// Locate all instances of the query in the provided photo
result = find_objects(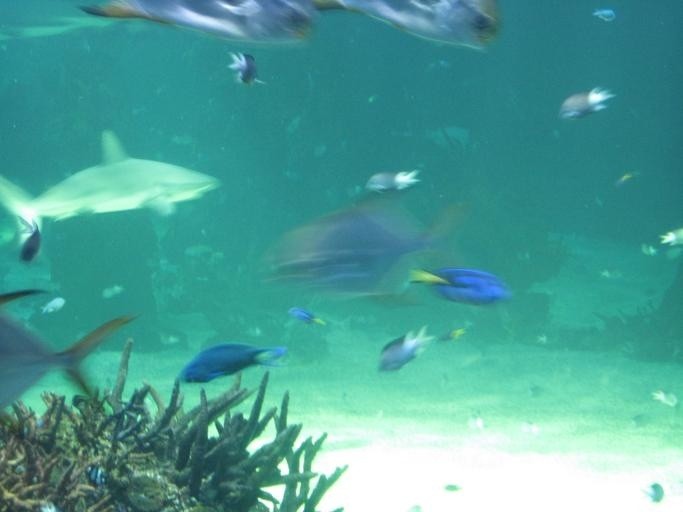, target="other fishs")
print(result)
[81,0,501,49]
[265,210,516,378]
